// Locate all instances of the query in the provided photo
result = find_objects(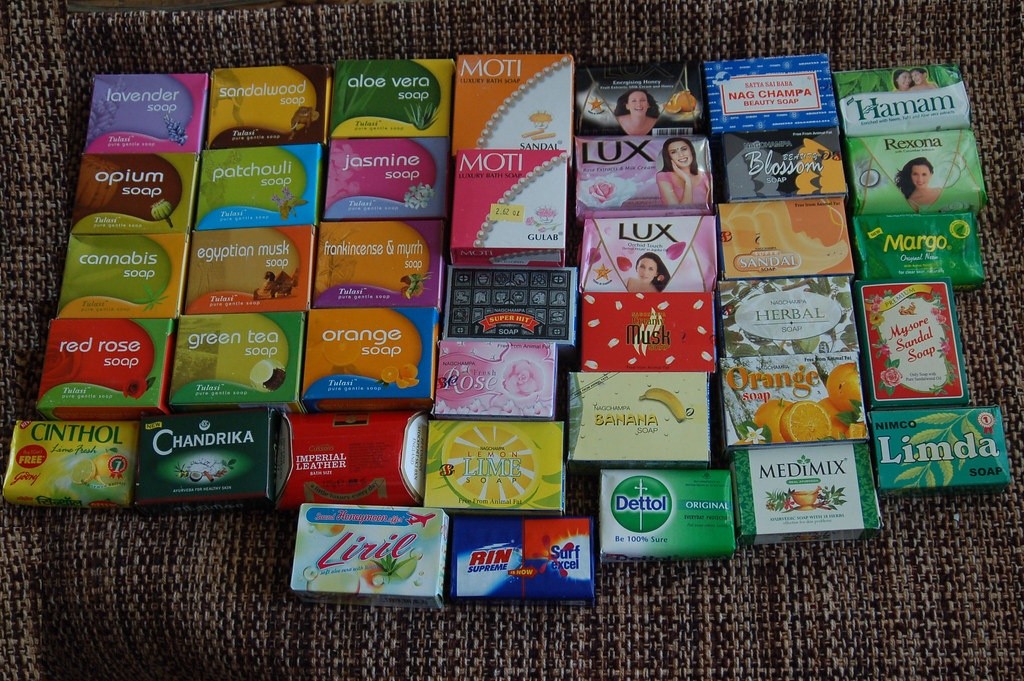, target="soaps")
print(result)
[2,52,1014,609]
